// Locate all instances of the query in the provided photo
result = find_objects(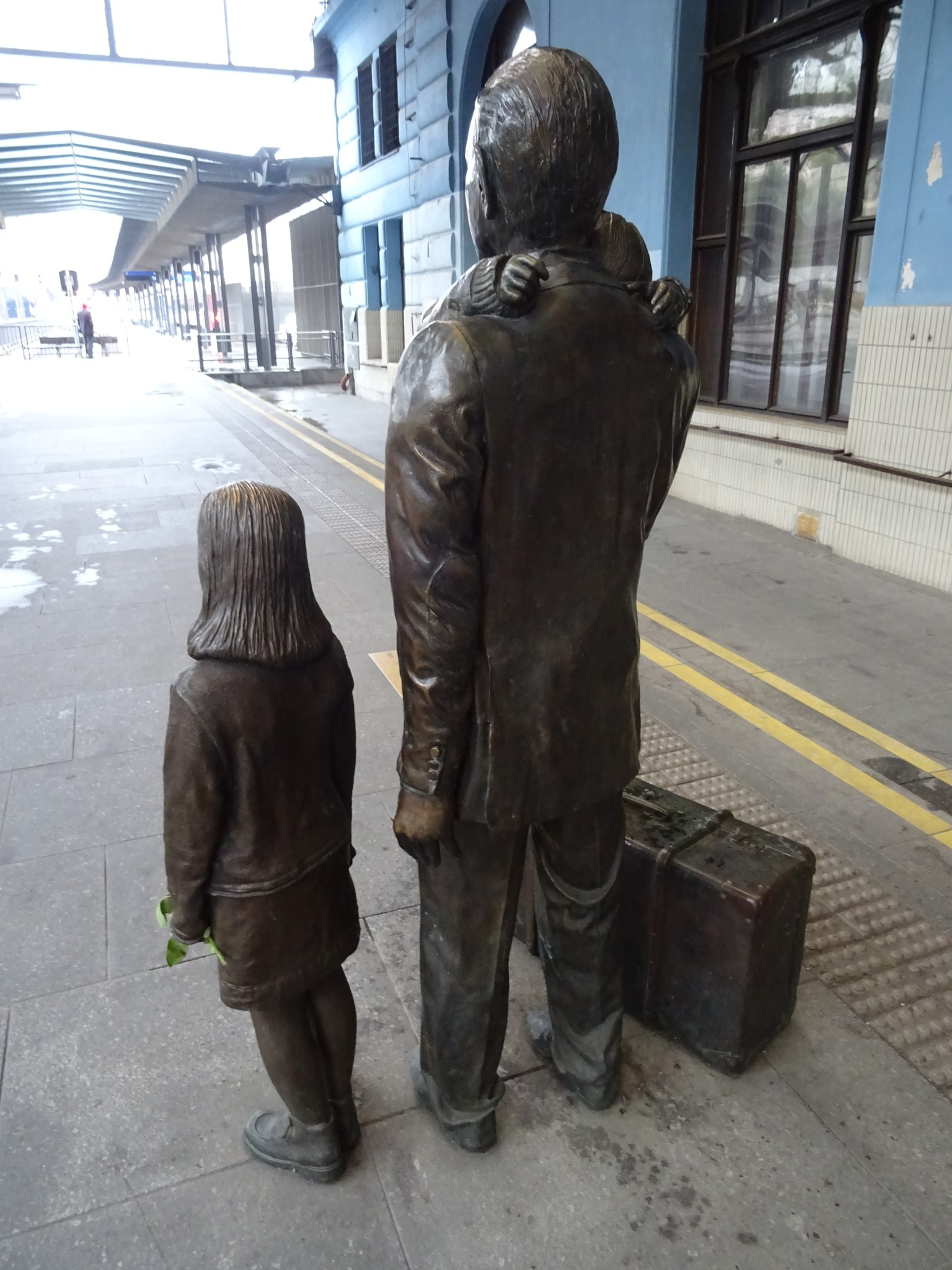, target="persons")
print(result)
[77,303,95,358]
[421,207,692,337]
[386,44,703,1152]
[160,480,365,1184]
